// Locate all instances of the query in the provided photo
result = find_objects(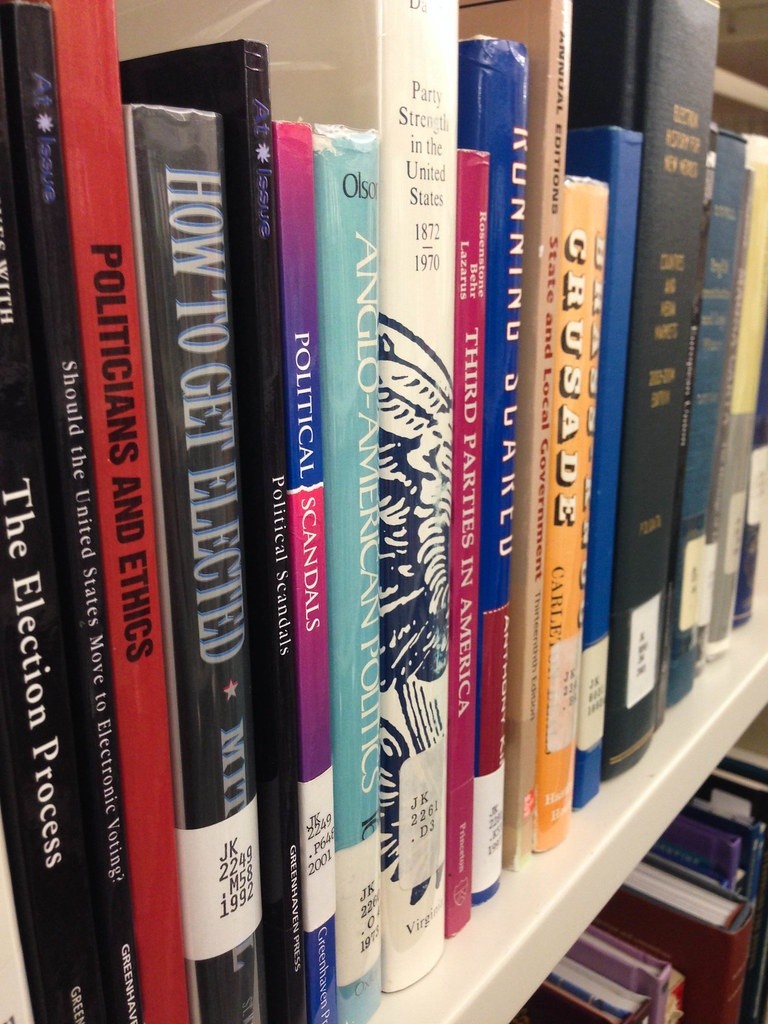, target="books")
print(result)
[0,0,768,1024]
